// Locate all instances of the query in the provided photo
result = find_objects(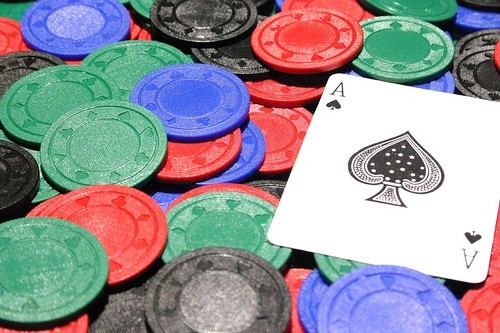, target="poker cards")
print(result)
[266,73,500,284]
